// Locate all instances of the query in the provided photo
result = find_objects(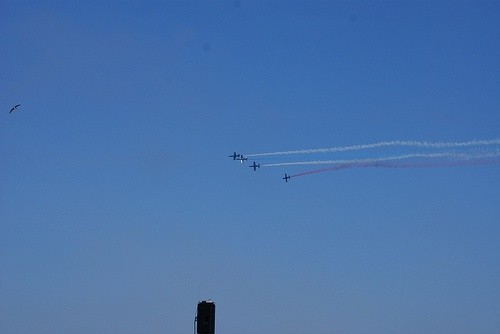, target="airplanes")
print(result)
[237,155,248,165]
[282,173,291,182]
[229,151,241,161]
[249,161,261,172]
